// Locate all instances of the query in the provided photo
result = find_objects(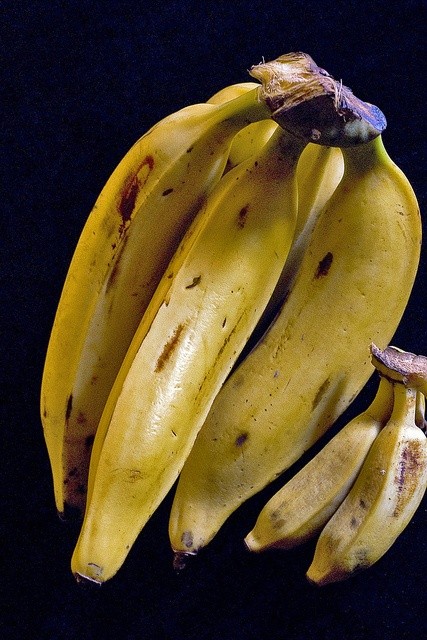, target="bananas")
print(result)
[242,343,427,585]
[39,51,421,587]
